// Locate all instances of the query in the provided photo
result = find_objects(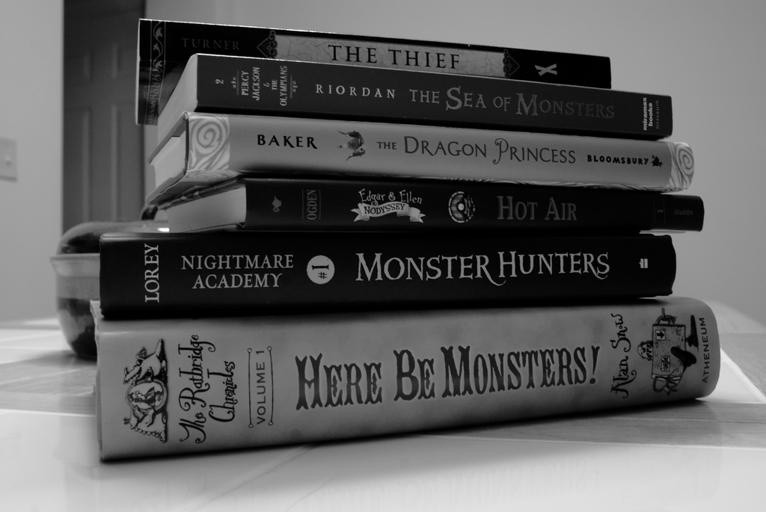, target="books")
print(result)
[81,15,723,464]
